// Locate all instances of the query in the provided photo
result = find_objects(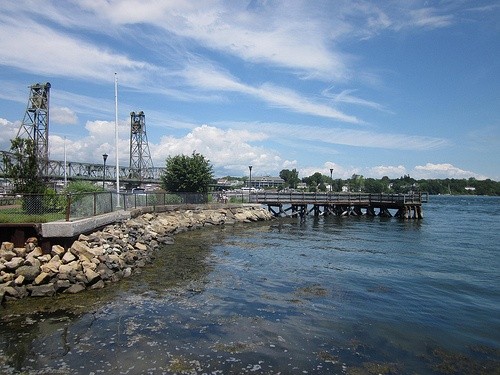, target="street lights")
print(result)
[249,165,253,203]
[113,71,123,210]
[102,154,108,190]
[330,168,334,192]
